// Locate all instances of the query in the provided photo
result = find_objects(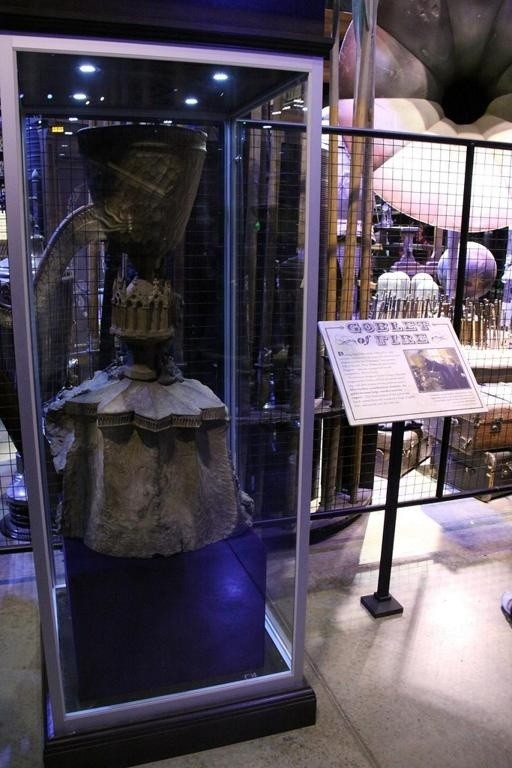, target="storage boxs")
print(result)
[375,382,512,503]
